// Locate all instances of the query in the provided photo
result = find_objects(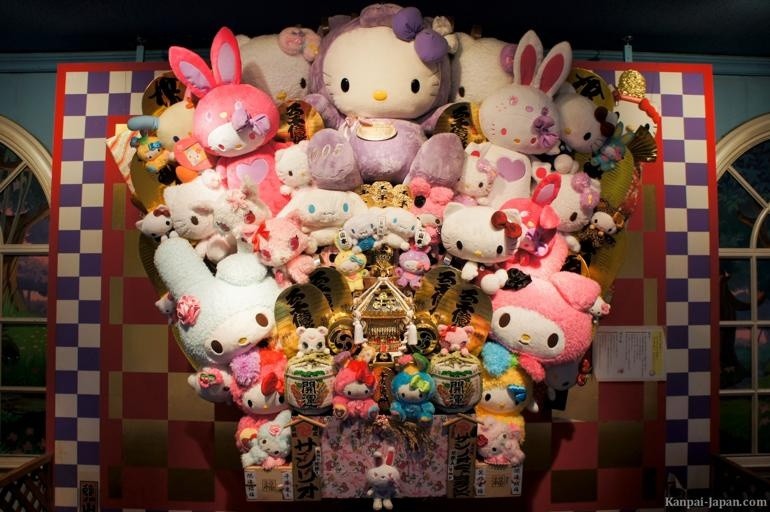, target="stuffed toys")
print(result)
[126,3,659,510]
